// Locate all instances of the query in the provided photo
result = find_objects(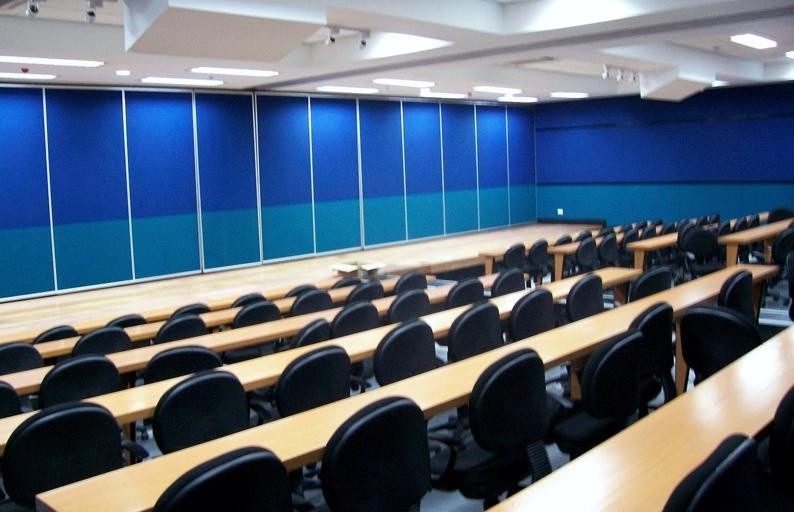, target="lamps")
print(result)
[16,0,108,28]
[601,65,637,88]
[326,26,370,51]
